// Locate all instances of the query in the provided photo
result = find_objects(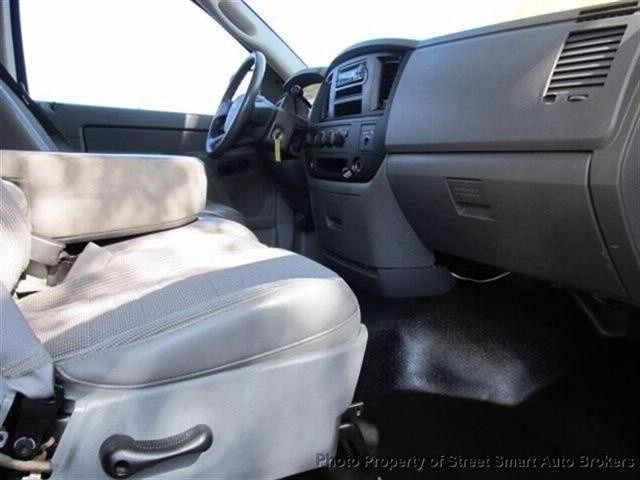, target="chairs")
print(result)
[1,186,379,479]
[0,63,247,289]
